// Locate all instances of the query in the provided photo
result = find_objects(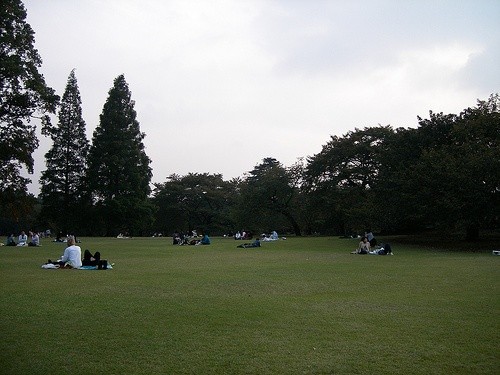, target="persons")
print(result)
[48,238,83,268]
[116,230,396,257]
[6,233,16,246]
[28,228,77,242]
[28,232,40,246]
[17,231,28,245]
[81,250,107,270]
